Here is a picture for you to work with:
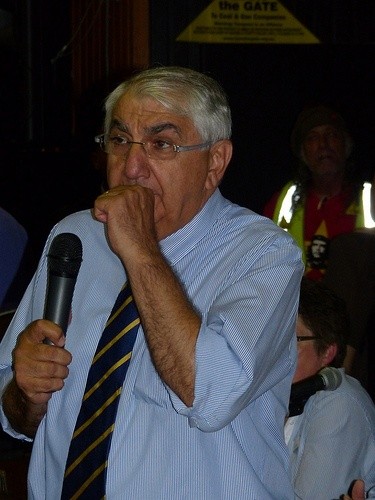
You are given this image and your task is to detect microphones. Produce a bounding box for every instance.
[42,233,83,351]
[285,367,344,418]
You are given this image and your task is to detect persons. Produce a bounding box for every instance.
[0,66,307,500]
[264,105,375,500]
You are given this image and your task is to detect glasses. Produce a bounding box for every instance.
[94,132,222,161]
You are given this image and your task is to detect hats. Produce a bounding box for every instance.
[291,102,345,158]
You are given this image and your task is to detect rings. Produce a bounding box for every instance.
[105,191,110,197]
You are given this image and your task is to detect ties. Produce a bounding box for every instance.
[60,278,142,500]
